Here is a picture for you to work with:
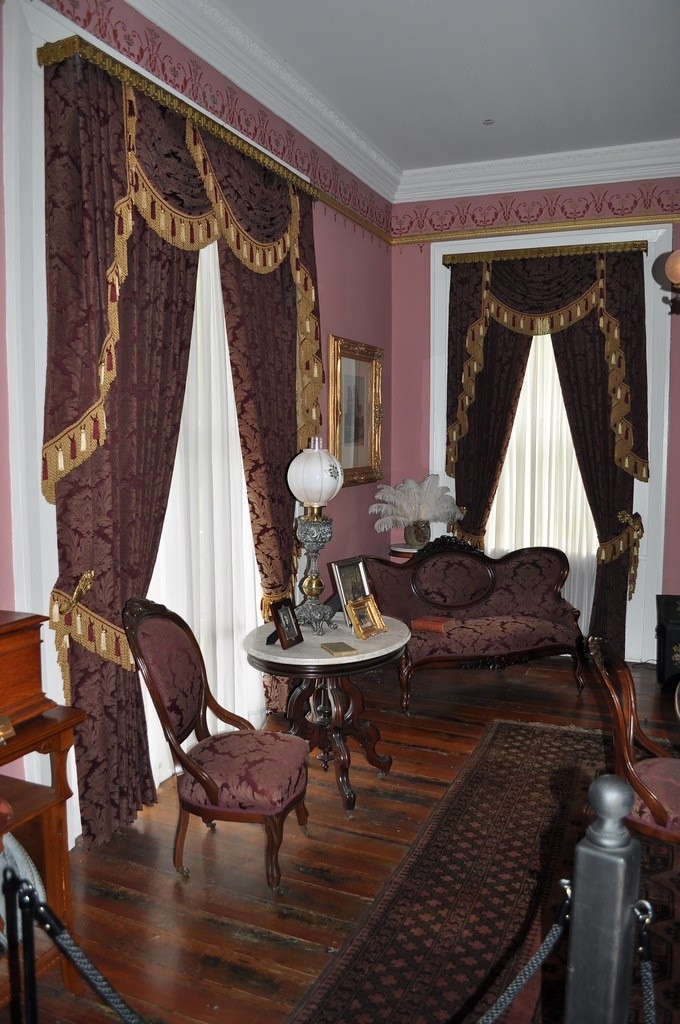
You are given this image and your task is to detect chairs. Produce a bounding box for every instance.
[581,635,680,845]
[122,598,310,896]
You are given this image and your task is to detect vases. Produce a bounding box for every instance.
[404,520,431,549]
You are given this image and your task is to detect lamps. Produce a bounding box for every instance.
[286,449,344,636]
[665,249,680,316]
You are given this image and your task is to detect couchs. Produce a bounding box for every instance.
[362,535,589,718]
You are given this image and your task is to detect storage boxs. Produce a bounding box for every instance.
[0,610,58,746]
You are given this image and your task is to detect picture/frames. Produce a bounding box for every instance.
[345,594,389,640]
[327,334,385,489]
[266,598,304,650]
[330,555,381,628]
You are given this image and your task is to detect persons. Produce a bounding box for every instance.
[344,384,364,442]
[350,576,363,599]
[355,609,369,625]
[282,613,295,639]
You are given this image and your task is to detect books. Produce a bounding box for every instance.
[411,615,456,633]
[322,641,357,656]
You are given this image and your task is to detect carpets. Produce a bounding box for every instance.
[282,719,680,1024]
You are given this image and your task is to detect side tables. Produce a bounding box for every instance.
[388,542,430,558]
[0,703,87,1011]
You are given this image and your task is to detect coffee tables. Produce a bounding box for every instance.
[242,611,411,821]
